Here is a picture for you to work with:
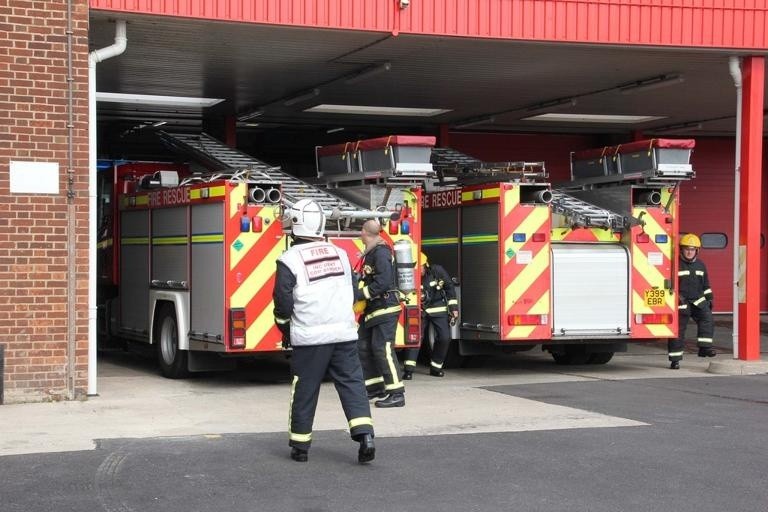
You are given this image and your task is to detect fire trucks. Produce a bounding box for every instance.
[421,137,697,371]
[97,128,439,381]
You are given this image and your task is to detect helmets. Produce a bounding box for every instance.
[680,233,701,248]
[421,252,428,265]
[290,199,326,238]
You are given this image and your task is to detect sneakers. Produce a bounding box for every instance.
[359,434,375,462]
[699,350,715,356]
[430,367,443,376]
[402,372,412,380]
[368,388,386,398]
[292,448,307,462]
[375,393,405,407]
[671,361,679,368]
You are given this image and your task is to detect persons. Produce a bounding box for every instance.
[352,218,406,410]
[402,252,458,379]
[272,197,377,464]
[667,234,717,369]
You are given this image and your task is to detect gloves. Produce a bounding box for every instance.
[358,288,364,300]
[274,318,290,348]
